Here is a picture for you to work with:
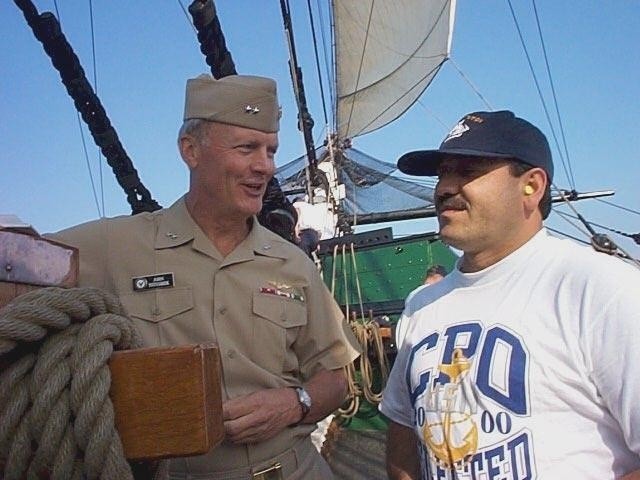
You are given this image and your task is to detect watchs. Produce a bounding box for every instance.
[287,383,313,430]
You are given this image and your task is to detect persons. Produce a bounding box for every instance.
[425,263,445,284]
[376,110,640,480]
[40,72,366,479]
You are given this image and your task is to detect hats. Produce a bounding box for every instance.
[182,72,283,134]
[395,108,555,187]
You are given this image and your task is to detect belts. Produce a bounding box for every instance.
[161,433,314,480]
